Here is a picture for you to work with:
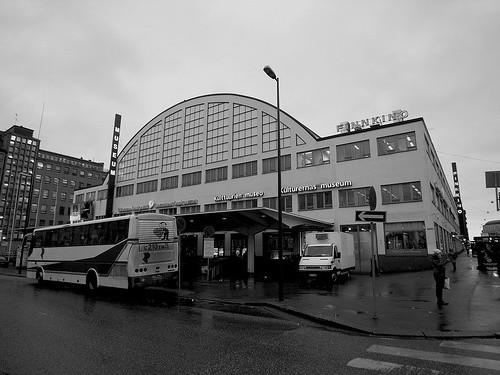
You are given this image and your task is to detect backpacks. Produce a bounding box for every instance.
[451,252,457,260]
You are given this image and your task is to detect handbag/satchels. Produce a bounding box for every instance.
[442,278,451,290]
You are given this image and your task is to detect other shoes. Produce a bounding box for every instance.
[436,301,443,310]
[440,300,449,305]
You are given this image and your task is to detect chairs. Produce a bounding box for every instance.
[58,236,122,246]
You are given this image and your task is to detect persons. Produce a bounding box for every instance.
[432,249,450,305]
[447,248,457,272]
[467,242,470,255]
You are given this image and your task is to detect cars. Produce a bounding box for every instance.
[0,256,8,268]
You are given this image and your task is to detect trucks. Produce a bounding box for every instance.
[296,230,356,283]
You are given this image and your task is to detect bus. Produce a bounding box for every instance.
[14,233,32,268]
[22,213,180,297]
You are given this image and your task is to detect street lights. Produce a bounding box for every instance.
[261,64,284,302]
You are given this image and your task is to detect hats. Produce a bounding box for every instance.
[434,249,442,254]
